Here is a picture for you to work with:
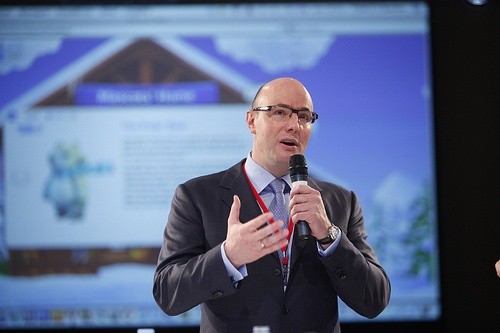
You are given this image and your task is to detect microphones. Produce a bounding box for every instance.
[289,153,311,241]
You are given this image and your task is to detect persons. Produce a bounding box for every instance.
[153,77,391,333]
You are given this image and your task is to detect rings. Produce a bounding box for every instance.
[260,242,265,249]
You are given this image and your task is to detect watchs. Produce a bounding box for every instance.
[317,223,340,244]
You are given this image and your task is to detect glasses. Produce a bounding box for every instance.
[253,106,318,124]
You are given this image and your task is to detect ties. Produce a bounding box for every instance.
[265,179,290,273]
